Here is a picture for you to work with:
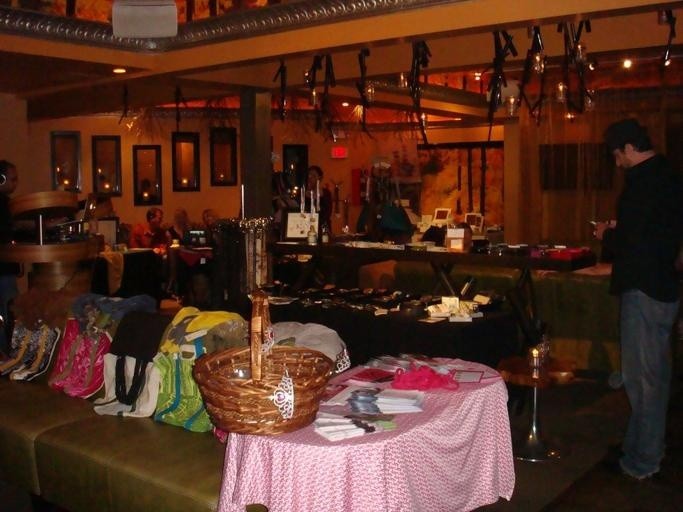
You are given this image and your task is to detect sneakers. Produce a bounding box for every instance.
[8,318,62,387]
[0,317,36,380]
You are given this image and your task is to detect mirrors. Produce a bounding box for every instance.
[282,144,308,195]
[50,127,237,206]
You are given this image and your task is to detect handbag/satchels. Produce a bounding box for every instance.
[151,343,219,434]
[63,280,248,361]
[91,352,163,422]
[44,315,115,405]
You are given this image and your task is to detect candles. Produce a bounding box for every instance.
[241,184,246,222]
[299,180,321,218]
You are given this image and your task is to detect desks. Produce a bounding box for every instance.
[271,286,512,373]
[218,354,517,512]
[499,355,574,463]
[276,245,598,345]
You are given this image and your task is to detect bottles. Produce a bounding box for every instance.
[528,346,549,382]
[170,239,181,248]
[307,225,317,246]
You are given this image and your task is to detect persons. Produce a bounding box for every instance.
[305,165,333,237]
[200,207,236,253]
[125,207,167,250]
[583,116,683,484]
[162,205,198,297]
[0,158,23,355]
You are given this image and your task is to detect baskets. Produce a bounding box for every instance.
[190,292,334,436]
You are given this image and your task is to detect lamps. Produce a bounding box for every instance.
[274,10,676,132]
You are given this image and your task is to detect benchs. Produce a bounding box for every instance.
[34,413,226,512]
[0,375,94,512]
